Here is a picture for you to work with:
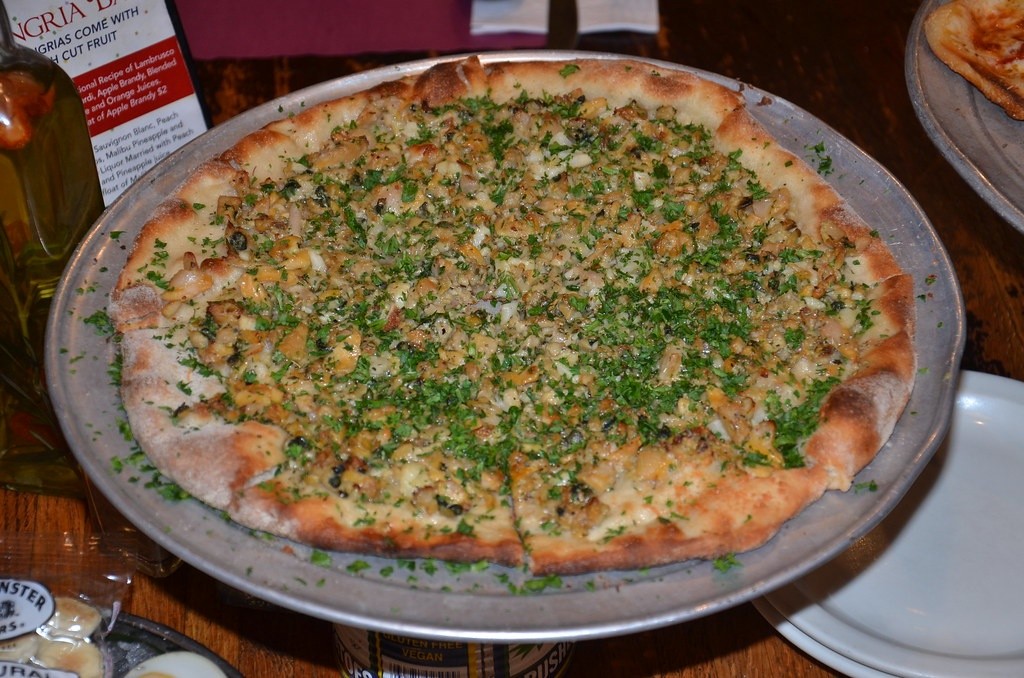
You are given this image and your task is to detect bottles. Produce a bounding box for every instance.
[2,0,205,577]
[328,621,573,677]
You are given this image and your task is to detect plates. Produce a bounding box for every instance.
[749,368,1021,678]
[904,0,1023,239]
[95,610,241,678]
[44,48,967,642]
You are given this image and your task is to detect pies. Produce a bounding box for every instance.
[925,0,1022,123]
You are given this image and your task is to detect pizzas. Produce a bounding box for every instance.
[108,55,918,576]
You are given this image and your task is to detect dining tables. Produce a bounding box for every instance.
[0,40,1021,678]
